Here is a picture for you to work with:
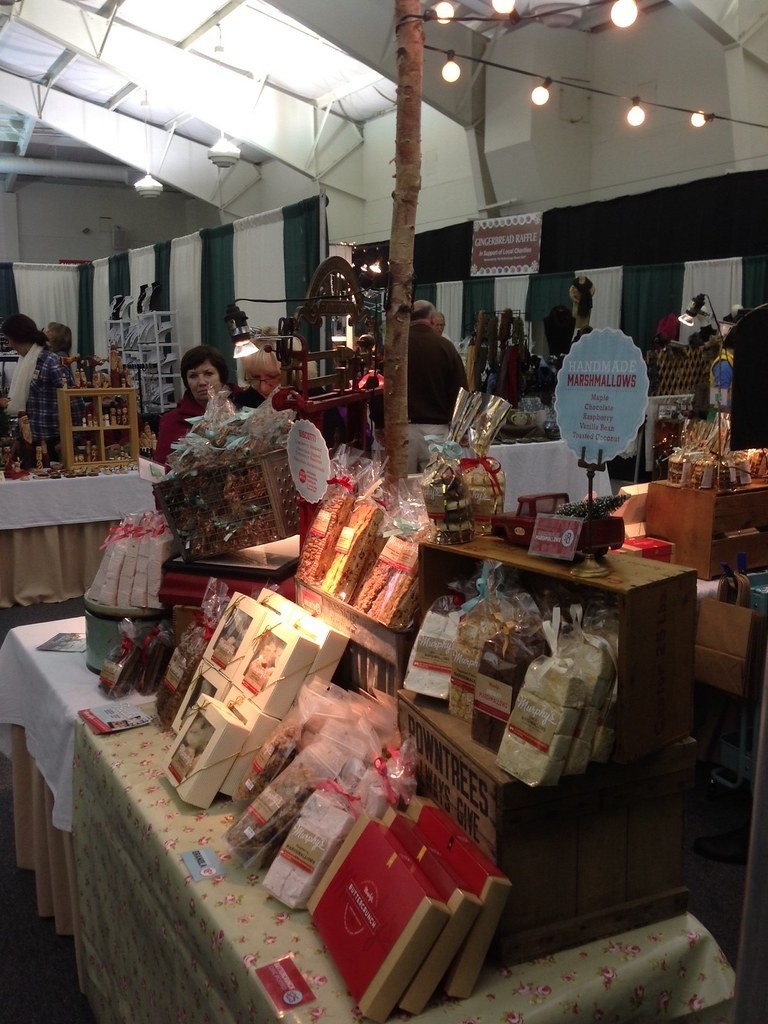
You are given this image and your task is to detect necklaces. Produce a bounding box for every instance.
[108,284,174,401]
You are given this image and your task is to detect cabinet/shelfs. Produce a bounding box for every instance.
[56,387,139,474]
[104,308,182,414]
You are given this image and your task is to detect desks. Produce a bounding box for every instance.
[410,424,612,513]
[0,469,156,609]
[0,616,736,1024]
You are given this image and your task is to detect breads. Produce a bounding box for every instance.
[298,492,423,632]
[424,466,505,545]
[449,613,619,787]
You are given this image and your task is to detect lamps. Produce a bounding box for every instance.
[134,89,163,199]
[360,256,383,273]
[207,22,241,168]
[677,292,708,328]
[222,303,259,359]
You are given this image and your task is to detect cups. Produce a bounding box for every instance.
[510,409,529,426]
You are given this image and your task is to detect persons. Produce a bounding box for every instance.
[0,313,85,461]
[706,349,734,424]
[228,324,348,449]
[45,322,72,374]
[569,272,595,328]
[369,300,471,474]
[435,311,445,335]
[155,344,244,473]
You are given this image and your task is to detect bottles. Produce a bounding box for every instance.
[140,447,153,461]
[82,413,99,427]
[104,407,130,425]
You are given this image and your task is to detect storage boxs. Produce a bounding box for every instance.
[152,446,301,563]
[610,482,649,540]
[294,534,697,1022]
[644,475,768,580]
